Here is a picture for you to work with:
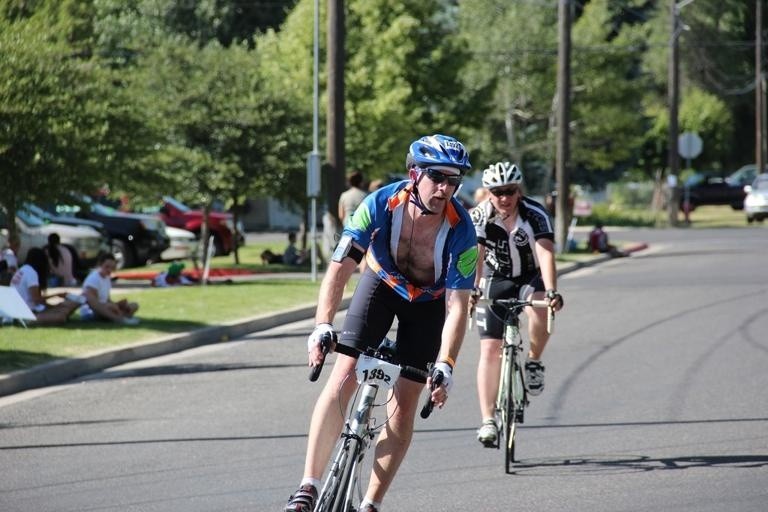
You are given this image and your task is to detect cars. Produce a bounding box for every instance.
[604,163,768,225]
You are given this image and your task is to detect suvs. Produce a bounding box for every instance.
[1,186,247,279]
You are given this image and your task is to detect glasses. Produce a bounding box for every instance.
[416,167,461,186]
[490,189,519,195]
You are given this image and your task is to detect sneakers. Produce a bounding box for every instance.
[476,418,499,442]
[118,315,141,326]
[357,502,380,512]
[524,354,545,396]
[284,484,319,512]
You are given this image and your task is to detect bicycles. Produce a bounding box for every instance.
[467,285,564,475]
[304,334,448,510]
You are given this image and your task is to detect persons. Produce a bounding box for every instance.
[283,232,327,268]
[0,234,140,325]
[467,161,564,447]
[589,223,629,258]
[283,134,477,512]
[368,179,383,193]
[337,170,368,228]
[261,250,284,265]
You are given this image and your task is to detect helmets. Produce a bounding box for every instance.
[481,161,524,191]
[404,133,471,171]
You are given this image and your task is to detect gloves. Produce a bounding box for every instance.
[431,359,453,398]
[544,290,565,309]
[307,323,336,351]
